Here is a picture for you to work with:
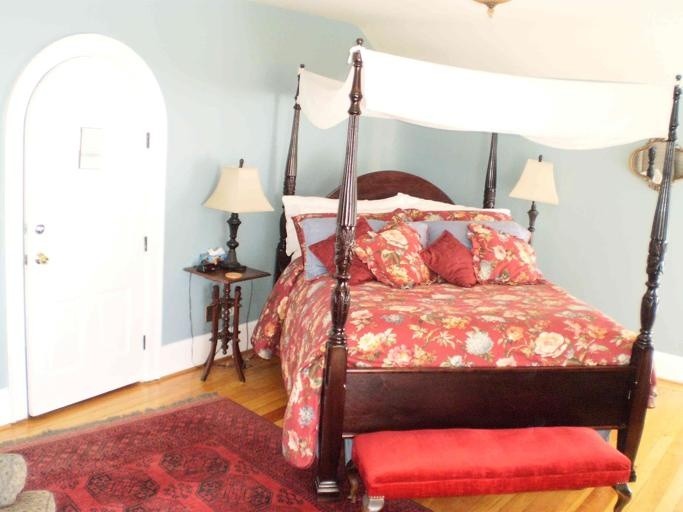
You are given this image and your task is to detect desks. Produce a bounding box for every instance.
[182,265,271,386]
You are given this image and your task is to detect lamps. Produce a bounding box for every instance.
[505,152,559,241]
[200,157,274,270]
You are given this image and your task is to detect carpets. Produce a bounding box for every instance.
[2,392,432,512]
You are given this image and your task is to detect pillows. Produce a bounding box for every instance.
[280,189,541,287]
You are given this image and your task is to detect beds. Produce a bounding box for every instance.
[250,37,683,497]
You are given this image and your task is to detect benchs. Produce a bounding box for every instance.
[346,426,634,512]
